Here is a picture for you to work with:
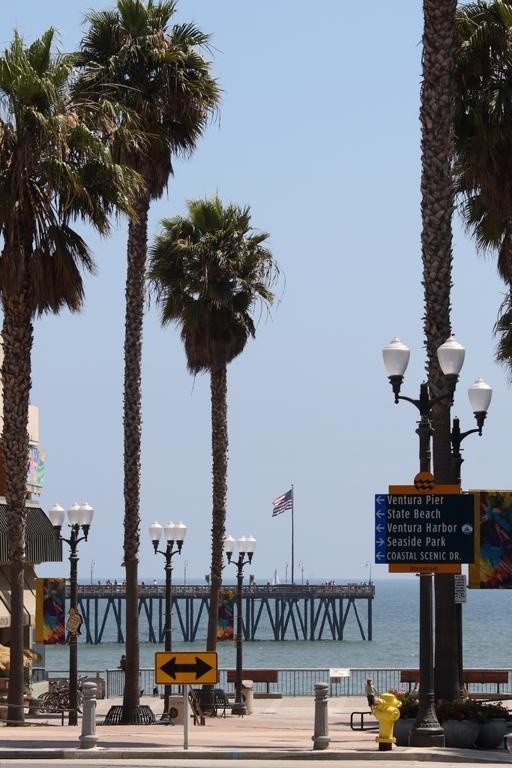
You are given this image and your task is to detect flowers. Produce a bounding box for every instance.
[389,688,508,720]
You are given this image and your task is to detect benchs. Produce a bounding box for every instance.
[189,688,247,718]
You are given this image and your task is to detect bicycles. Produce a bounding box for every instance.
[33,674,89,716]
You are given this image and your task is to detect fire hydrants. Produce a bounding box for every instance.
[370,691,403,752]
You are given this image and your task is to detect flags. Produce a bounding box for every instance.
[272,488,293,518]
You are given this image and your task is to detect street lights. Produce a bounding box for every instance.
[378,329,468,745]
[47,501,95,727]
[146,519,186,722]
[429,377,492,731]
[89,558,95,585]
[222,533,258,717]
[366,559,372,586]
[297,559,304,585]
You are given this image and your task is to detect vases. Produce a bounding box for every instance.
[392,718,507,748]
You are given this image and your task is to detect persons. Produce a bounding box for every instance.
[364,679,377,710]
